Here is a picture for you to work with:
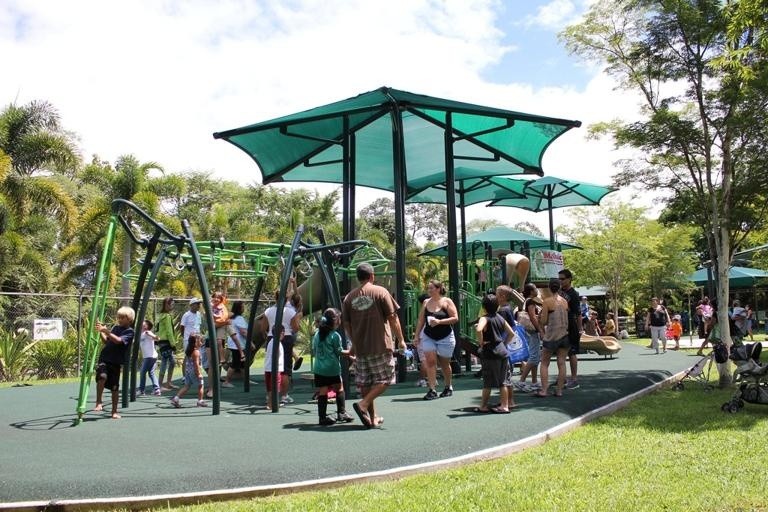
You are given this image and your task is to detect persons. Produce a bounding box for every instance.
[602,293,755,357]
[92,305,135,419]
[311,307,352,425]
[342,263,407,429]
[553,269,583,388]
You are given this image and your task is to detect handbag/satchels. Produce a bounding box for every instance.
[517,298,539,332]
[497,308,530,364]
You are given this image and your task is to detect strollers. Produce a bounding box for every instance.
[672,336,728,396]
[722,341,767,413]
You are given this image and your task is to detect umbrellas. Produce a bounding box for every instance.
[402,166,534,272]
[490,174,624,249]
[416,226,582,284]
[213,87,582,382]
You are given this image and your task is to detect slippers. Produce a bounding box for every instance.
[530,391,552,397]
[472,407,488,412]
[293,357,303,371]
[552,390,562,397]
[491,402,518,414]
[371,416,385,427]
[353,401,371,427]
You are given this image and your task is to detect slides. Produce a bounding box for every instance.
[223,265,330,379]
[575,333,622,360]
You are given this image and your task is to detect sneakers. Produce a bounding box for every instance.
[219,377,235,388]
[170,397,181,408]
[566,380,581,390]
[439,385,453,397]
[530,381,551,390]
[423,390,438,400]
[318,416,337,425]
[338,411,354,422]
[548,380,567,387]
[515,381,533,393]
[206,386,213,398]
[415,378,440,388]
[249,379,260,385]
[196,400,209,407]
[134,389,145,396]
[151,384,180,397]
[264,397,294,410]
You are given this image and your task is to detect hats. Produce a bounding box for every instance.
[671,314,681,321]
[189,297,204,305]
